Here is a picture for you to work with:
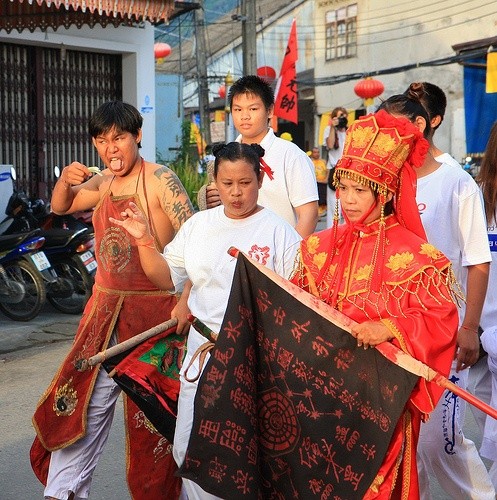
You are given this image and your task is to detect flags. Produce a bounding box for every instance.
[173,249,420,500]
[273,20,299,124]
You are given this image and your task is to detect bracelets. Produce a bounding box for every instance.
[460,325,479,333]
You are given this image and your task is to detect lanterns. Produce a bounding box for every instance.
[217,85,229,99]
[154,42,171,64]
[354,78,385,106]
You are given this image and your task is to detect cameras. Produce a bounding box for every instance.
[336,113,348,128]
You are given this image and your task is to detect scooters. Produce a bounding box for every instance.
[0,186,97,315]
[0,229,52,324]
[37,167,95,250]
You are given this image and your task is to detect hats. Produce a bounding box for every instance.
[333,107,415,194]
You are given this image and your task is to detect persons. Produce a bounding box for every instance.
[108,141,304,500]
[197,77,319,239]
[288,83,497,500]
[28,102,196,500]
